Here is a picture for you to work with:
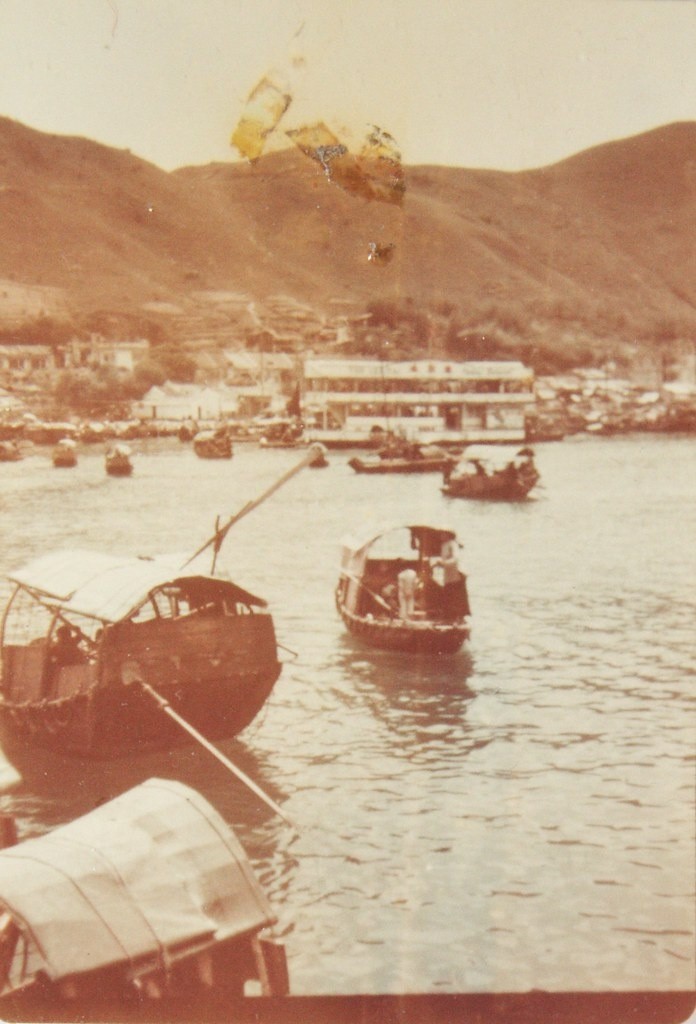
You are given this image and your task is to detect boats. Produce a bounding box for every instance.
[104,445,134,477]
[192,431,236,459]
[1,773,696,1024]
[440,444,541,499]
[0,441,24,460]
[50,439,78,467]
[1,547,281,761]
[261,355,563,446]
[336,511,475,653]
[348,451,458,473]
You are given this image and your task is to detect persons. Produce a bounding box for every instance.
[440,543,471,624]
[470,461,486,475]
[52,624,85,663]
[397,565,416,617]
